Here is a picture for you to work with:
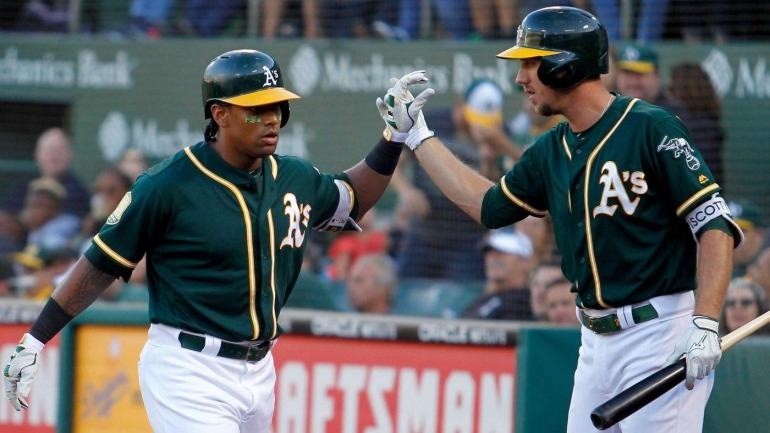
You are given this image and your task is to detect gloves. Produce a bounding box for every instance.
[383,70,435,143]
[3,333,45,411]
[662,314,723,390]
[376,78,435,152]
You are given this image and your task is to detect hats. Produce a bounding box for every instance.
[616,45,660,74]
[7,243,57,271]
[727,199,763,231]
[474,227,534,258]
[462,78,504,129]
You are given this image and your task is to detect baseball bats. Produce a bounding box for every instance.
[589,310,769,431]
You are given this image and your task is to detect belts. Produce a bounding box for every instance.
[179,331,273,363]
[578,304,659,335]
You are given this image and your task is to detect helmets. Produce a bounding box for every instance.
[495,6,609,90]
[201,49,301,128]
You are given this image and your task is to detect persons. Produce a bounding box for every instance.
[0,0,770,338]
[375,7,745,433]
[2,48,435,433]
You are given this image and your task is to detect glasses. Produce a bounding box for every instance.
[724,300,757,308]
